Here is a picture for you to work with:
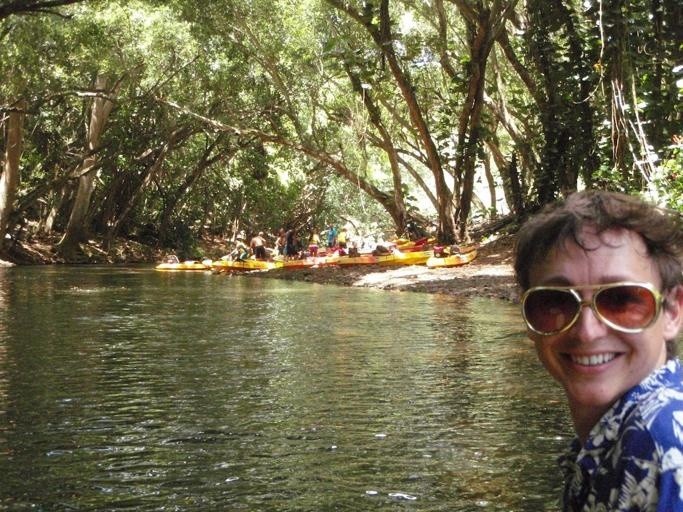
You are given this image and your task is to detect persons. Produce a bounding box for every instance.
[221,224,348,265]
[511,192,682,512]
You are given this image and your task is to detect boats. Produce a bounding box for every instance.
[156,259,208,270]
[200,237,481,280]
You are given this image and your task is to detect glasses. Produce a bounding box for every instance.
[521,281,665,335]
